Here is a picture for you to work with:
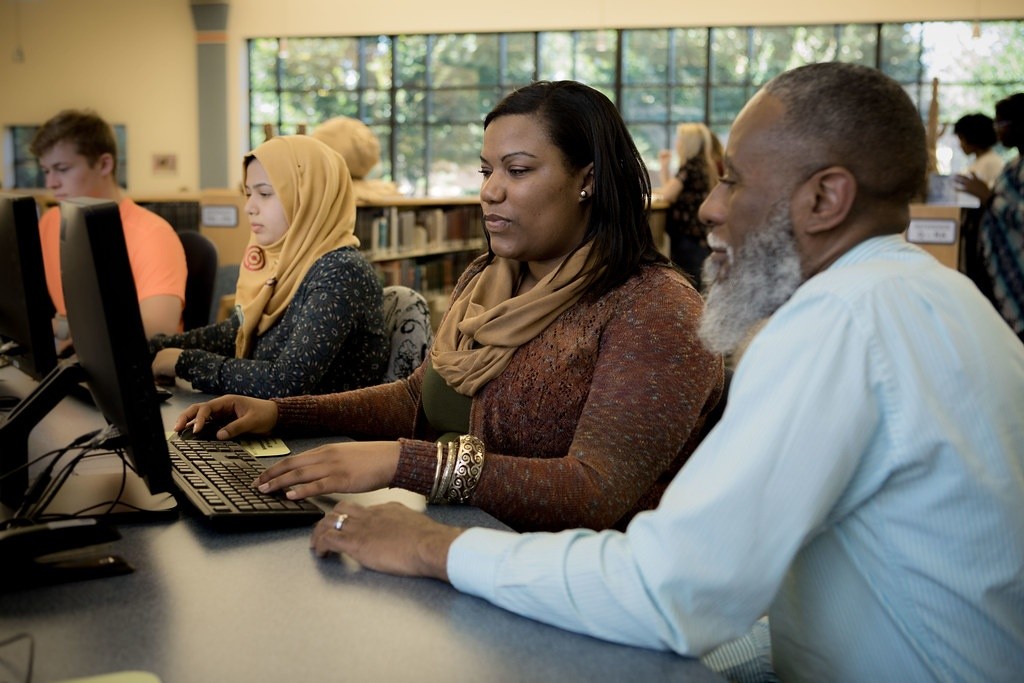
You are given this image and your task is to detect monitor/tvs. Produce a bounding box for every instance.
[0,194,175,505]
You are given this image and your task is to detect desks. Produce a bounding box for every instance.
[1,341,732,683]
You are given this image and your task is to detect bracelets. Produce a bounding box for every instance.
[426,434,485,510]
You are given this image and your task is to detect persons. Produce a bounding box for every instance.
[32,109,187,365]
[150,135,390,408]
[312,59,1024,683]
[951,92,1024,346]
[651,122,725,296]
[172,80,727,562]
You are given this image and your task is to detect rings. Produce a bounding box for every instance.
[335,514,349,530]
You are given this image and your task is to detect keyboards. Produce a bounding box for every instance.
[165,439,327,530]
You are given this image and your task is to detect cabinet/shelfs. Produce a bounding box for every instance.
[351,196,490,333]
